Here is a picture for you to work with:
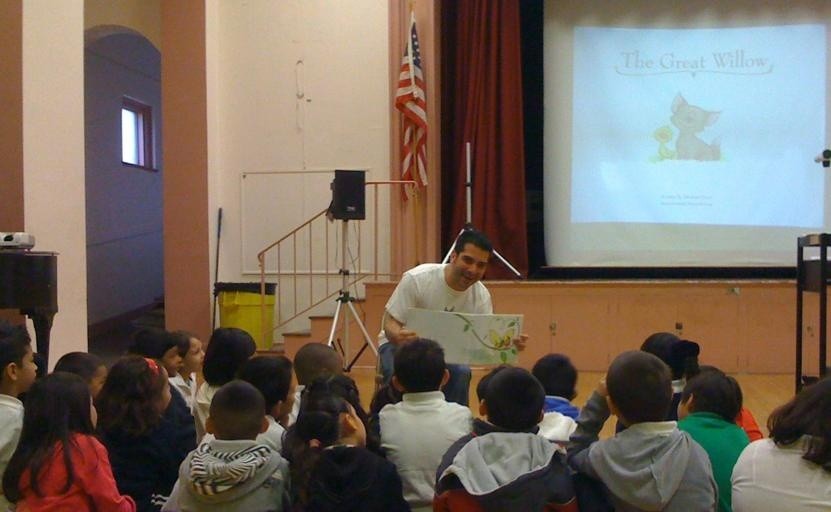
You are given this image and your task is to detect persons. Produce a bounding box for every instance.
[0,322,37,512]
[91,358,174,512]
[734,404,764,444]
[194,355,289,458]
[615,333,702,433]
[131,333,198,451]
[295,342,347,387]
[530,354,582,444]
[370,231,530,455]
[676,367,753,512]
[282,393,409,512]
[433,368,578,511]
[160,382,291,512]
[281,374,382,464]
[1,372,137,512]
[194,327,261,448]
[731,372,831,511]
[379,339,477,512]
[566,351,720,511]
[167,331,207,417]
[52,352,109,403]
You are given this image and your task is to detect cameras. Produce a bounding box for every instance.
[330,169,367,219]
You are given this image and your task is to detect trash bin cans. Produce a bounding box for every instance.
[213,282,277,351]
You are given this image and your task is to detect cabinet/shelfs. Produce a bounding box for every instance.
[1,251,58,375]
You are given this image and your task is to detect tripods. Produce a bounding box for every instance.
[443,144,519,280]
[324,220,380,370]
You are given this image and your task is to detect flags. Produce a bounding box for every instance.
[395,10,429,213]
